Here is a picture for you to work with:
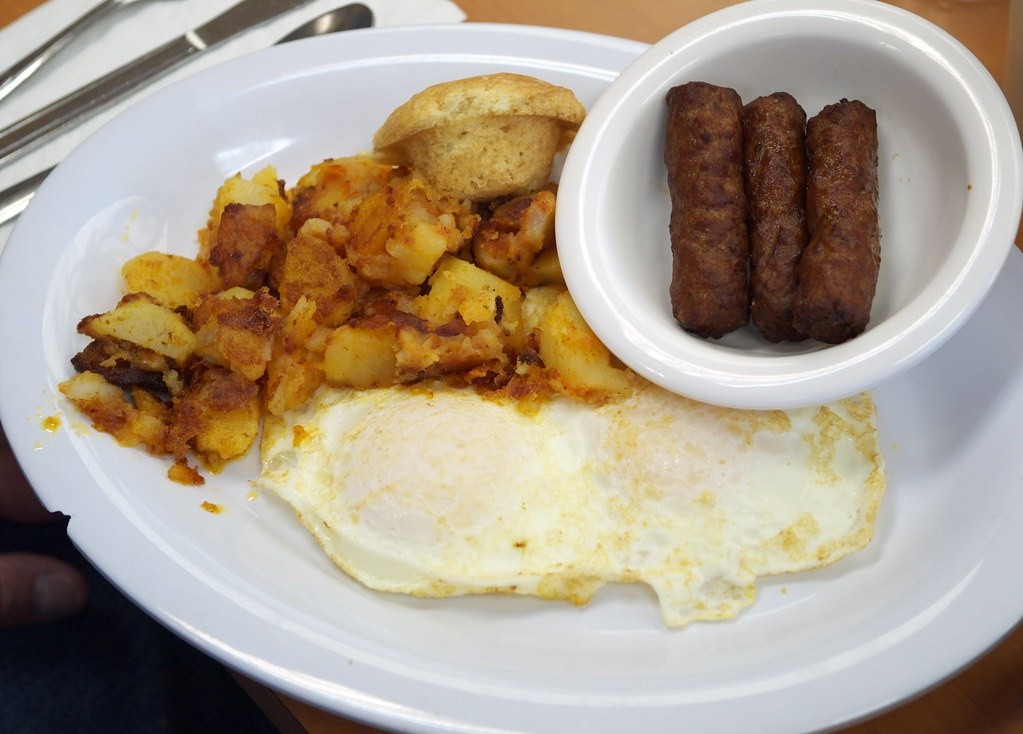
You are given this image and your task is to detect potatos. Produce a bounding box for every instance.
[59,153,622,484]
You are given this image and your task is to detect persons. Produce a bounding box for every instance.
[2,422,281,734]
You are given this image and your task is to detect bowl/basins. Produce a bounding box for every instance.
[548,3,1023,409]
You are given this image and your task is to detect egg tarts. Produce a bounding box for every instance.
[372,74,588,206]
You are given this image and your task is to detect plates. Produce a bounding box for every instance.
[0,20,1022,733]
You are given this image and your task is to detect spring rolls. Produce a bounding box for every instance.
[664,80,883,346]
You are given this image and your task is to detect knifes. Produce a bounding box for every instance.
[0,0,315,158]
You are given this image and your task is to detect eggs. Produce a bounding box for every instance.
[255,370,889,628]
